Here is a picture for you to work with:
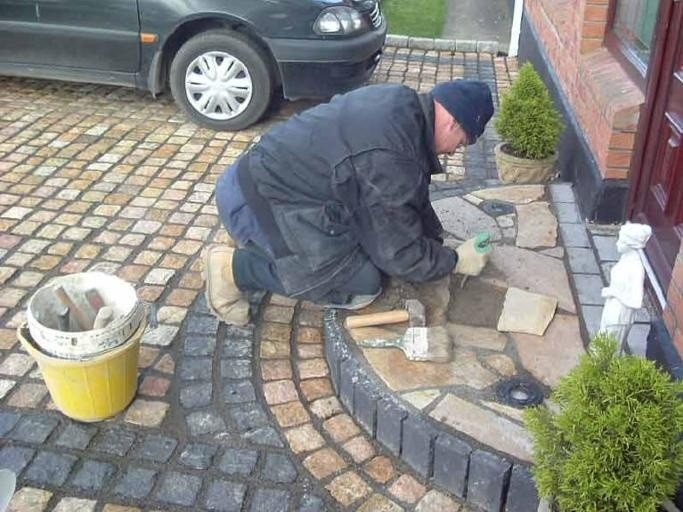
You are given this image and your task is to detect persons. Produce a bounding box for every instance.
[598,222,652,359]
[200,76,495,329]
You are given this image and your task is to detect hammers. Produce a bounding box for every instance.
[347,300,426,328]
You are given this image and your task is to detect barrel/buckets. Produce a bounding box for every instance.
[17,322,147,422]
[28,270,144,357]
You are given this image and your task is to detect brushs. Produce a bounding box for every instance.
[356,326,453,363]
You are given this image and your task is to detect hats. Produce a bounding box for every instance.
[428,76,497,150]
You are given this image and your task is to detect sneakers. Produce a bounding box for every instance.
[198,241,255,330]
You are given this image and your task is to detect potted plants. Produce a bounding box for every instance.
[520,329,683,512]
[494,59,568,186]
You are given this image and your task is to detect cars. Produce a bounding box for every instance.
[0,1,387,131]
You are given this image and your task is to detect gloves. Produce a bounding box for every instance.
[448,230,494,280]
